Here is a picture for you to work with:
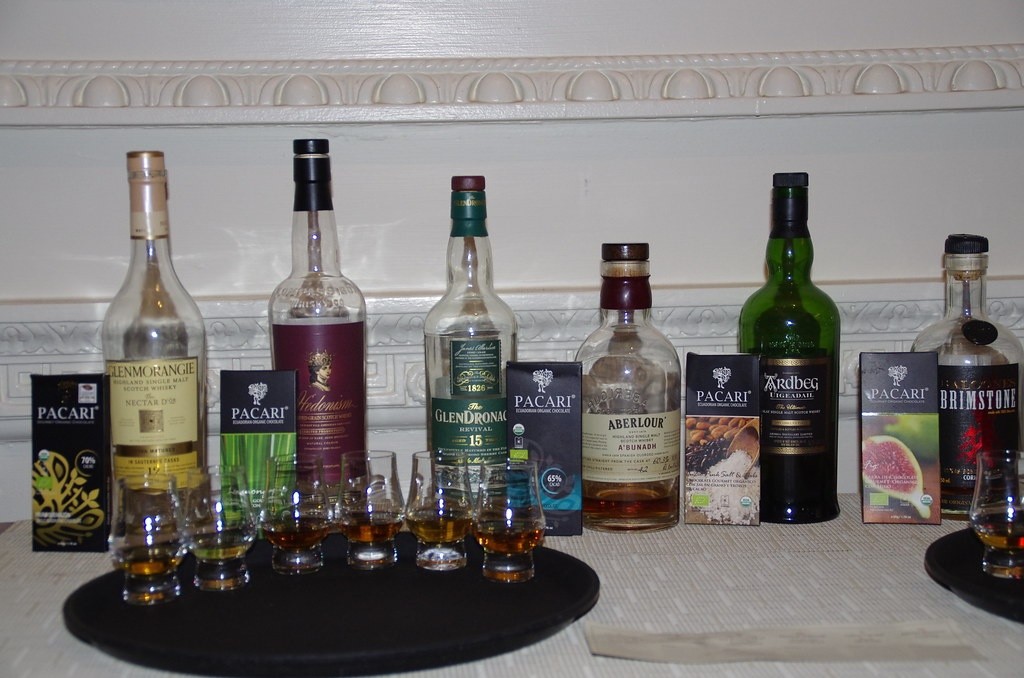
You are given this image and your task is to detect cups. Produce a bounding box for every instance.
[472,459,545,583]
[109,474,186,604]
[970,450,1024,579]
[334,450,405,571]
[184,465,257,591]
[258,454,333,575]
[405,450,471,573]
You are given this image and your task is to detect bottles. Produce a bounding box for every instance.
[103,152,213,548]
[423,176,518,521]
[738,173,840,524]
[269,139,366,534]
[575,244,680,532]
[909,234,1024,514]
